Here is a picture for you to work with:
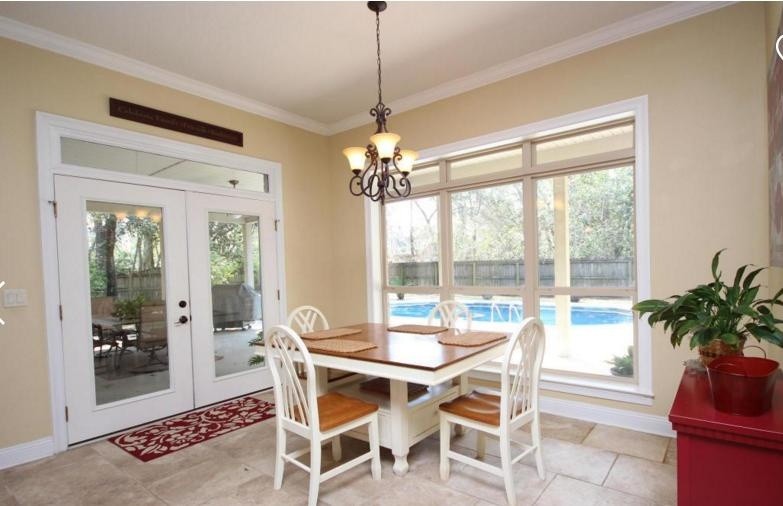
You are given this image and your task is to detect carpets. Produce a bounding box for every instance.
[106,395,277,463]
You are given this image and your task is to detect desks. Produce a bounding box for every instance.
[254,324,524,478]
[91,310,169,371]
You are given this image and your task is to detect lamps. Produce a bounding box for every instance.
[342,1,420,207]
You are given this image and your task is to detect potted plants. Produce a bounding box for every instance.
[631,247,783,369]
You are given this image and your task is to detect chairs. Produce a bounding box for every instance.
[113,302,168,376]
[284,305,368,407]
[90,296,133,361]
[435,315,546,506]
[426,300,472,330]
[262,324,382,506]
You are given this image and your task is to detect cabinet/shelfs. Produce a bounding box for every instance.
[665,358,783,505]
